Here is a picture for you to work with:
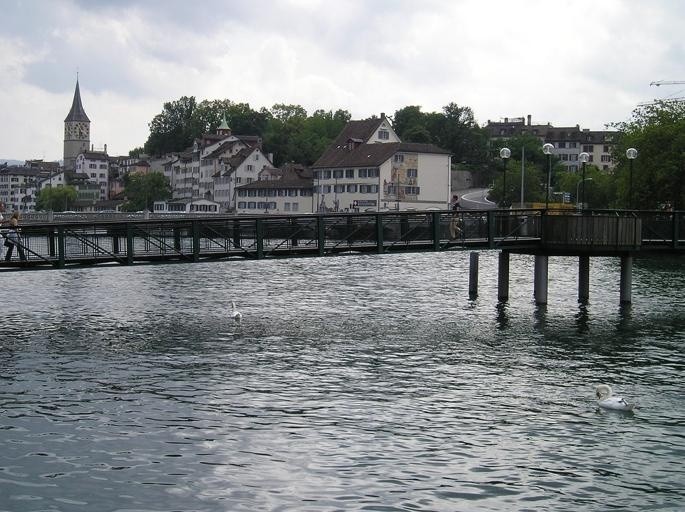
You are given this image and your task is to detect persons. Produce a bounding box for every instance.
[0,201,6,261]
[449,195,465,241]
[4,211,28,261]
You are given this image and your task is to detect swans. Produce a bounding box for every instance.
[596,385,635,412]
[230,301,242,320]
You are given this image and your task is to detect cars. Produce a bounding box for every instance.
[53,210,77,222]
[93,210,123,221]
[126,210,155,220]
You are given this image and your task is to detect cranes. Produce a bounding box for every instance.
[636,80,685,107]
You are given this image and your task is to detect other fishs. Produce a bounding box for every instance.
[596,391,603,399]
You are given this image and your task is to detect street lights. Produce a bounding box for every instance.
[578,152,590,210]
[500,148,511,210]
[626,148,638,210]
[542,143,555,208]
